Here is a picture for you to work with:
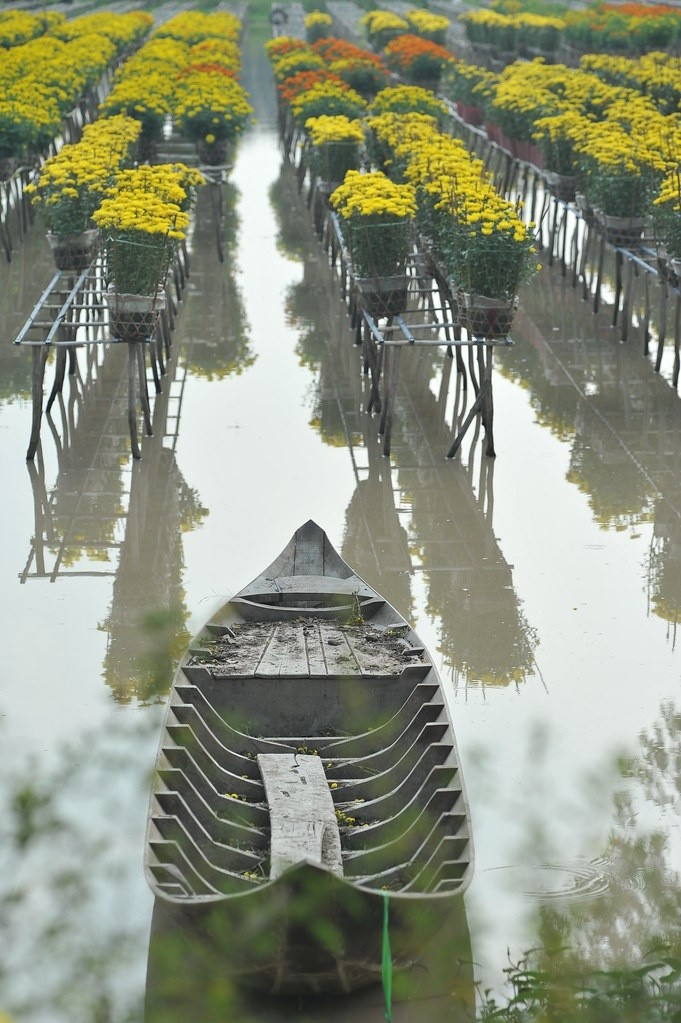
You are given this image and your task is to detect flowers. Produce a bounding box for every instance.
[0,0,681,301]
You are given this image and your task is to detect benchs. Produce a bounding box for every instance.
[233,575,380,603]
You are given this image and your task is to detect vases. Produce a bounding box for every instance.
[319,181,350,211]
[100,289,166,341]
[43,232,98,270]
[549,173,577,200]
[597,213,647,250]
[197,138,228,164]
[656,247,681,289]
[574,193,594,221]
[462,293,519,338]
[530,146,546,169]
[486,126,508,146]
[516,140,528,161]
[458,103,484,125]
[355,273,409,313]
